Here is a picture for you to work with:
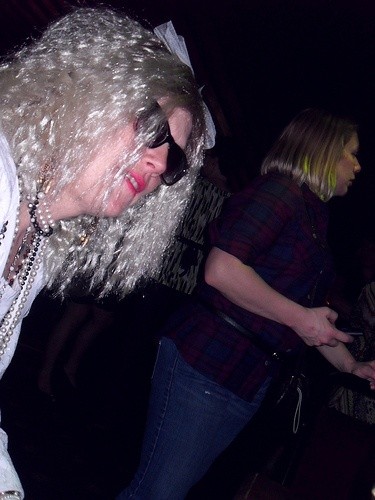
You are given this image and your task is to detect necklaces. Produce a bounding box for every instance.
[0,158,100,356]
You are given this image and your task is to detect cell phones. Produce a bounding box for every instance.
[340,327,364,335]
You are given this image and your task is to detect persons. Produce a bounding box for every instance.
[131,110,374,500]
[0,10,216,500]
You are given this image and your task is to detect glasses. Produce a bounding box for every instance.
[133,97,189,186]
